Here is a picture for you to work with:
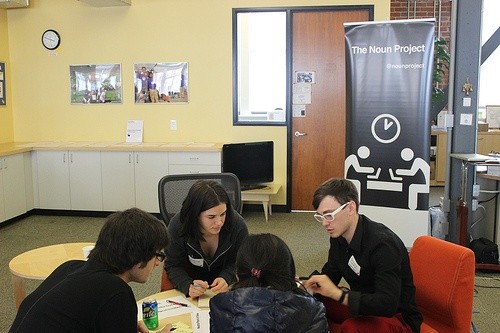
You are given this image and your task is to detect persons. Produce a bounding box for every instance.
[304,178,423,333]
[18,208,173,333]
[135,63,158,103]
[210,233,330,333]
[165,180,248,298]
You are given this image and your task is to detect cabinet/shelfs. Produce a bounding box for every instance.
[430,130,500,186]
[0,150,221,230]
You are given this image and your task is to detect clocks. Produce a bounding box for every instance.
[42,29,60,50]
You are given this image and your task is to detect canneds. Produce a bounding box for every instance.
[142,299,159,330]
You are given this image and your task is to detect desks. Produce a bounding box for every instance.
[9,242,95,310]
[226,182,282,223]
[137,287,230,333]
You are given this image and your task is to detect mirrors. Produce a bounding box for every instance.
[232,7,287,126]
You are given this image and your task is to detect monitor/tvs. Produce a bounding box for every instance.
[223,140,274,191]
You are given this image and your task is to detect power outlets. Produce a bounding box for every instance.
[170,120,177,130]
[472,200,479,211]
[473,184,480,197]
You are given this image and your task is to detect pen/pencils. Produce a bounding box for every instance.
[295,277,309,280]
[170,328,176,331]
[166,300,187,307]
[196,313,199,328]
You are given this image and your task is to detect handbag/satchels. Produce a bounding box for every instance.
[469,237,499,273]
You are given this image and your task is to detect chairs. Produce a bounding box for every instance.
[158,173,242,227]
[409,235,475,333]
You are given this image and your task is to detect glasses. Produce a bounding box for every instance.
[314,202,350,222]
[153,252,166,262]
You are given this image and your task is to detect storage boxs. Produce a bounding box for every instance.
[478,120,488,132]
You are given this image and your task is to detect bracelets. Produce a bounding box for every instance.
[338,292,346,303]
